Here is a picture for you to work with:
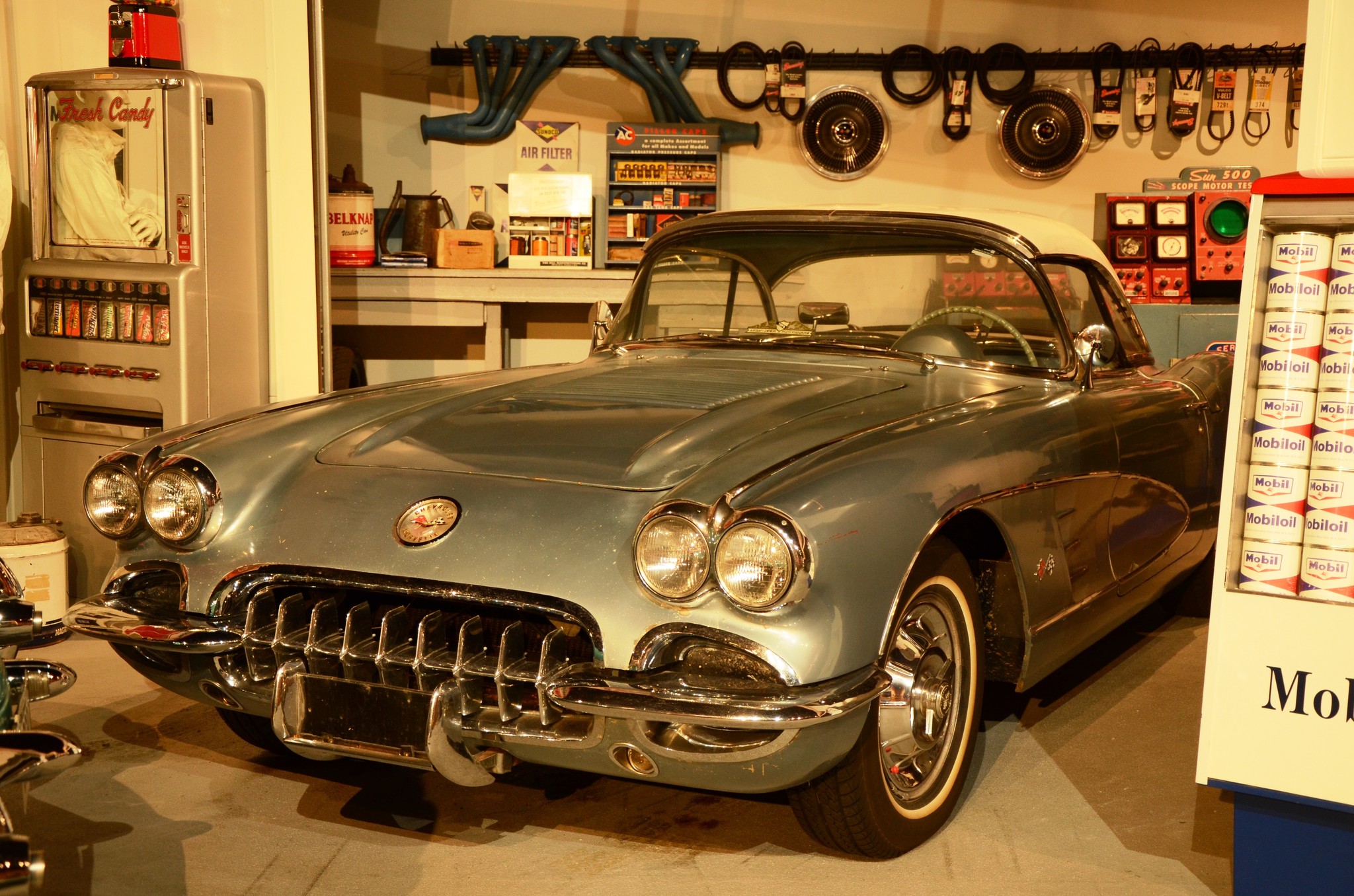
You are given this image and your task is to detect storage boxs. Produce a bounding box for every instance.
[428,225,498,272]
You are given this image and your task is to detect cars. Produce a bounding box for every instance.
[61,210,1234,863]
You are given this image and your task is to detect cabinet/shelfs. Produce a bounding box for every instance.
[599,120,728,268]
[503,177,594,272]
